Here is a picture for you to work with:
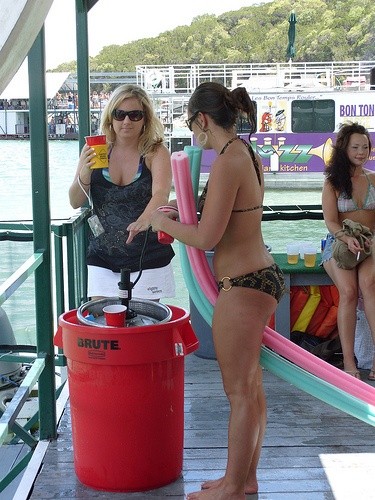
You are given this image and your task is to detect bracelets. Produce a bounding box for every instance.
[80,178,91,185]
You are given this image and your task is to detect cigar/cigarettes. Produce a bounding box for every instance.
[357,251,360,260]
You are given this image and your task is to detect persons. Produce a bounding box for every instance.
[69,84,175,303]
[151,82,285,500]
[318,120,375,380]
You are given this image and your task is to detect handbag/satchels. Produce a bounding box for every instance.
[333,219,373,269]
[291,330,358,369]
[351,309,375,370]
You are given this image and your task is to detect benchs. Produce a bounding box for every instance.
[270,252,335,340]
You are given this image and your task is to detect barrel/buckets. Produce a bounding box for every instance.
[190,244,272,360]
[53,304,200,493]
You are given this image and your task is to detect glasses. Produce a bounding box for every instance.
[112,109,144,121]
[185,112,205,132]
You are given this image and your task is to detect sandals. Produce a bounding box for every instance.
[368,370,375,381]
[344,370,361,382]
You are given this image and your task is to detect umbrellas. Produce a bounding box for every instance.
[286,13,298,80]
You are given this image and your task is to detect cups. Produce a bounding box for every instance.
[156,205,179,245]
[84,135,106,146]
[87,145,108,169]
[321,239,327,256]
[103,305,127,328]
[285,243,300,264]
[300,241,312,259]
[304,247,317,268]
